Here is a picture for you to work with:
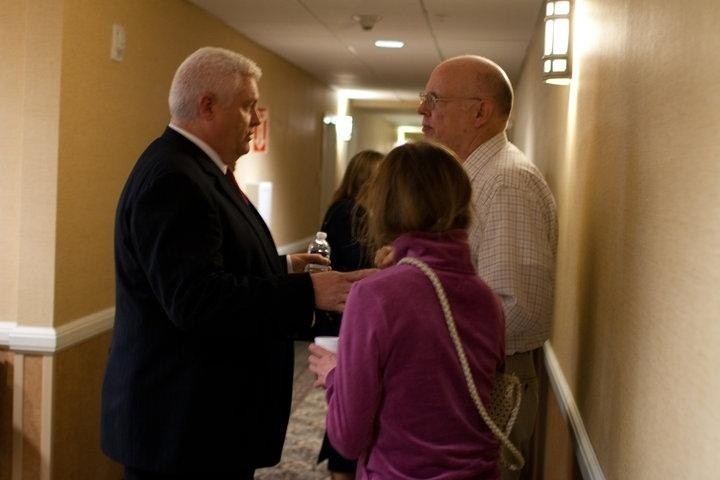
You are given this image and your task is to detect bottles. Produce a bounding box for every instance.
[306,231,331,273]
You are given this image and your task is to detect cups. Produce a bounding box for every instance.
[314,336,338,355]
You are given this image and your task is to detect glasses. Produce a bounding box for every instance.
[416,87,483,111]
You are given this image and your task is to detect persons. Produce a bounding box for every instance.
[418,55,559,480]
[308,138,505,480]
[102,46,380,480]
[307,150,385,342]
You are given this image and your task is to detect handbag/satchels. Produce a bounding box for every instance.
[483,370,525,442]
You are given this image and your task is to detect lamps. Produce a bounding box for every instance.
[537,0,576,86]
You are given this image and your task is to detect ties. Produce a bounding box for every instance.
[224,164,250,207]
